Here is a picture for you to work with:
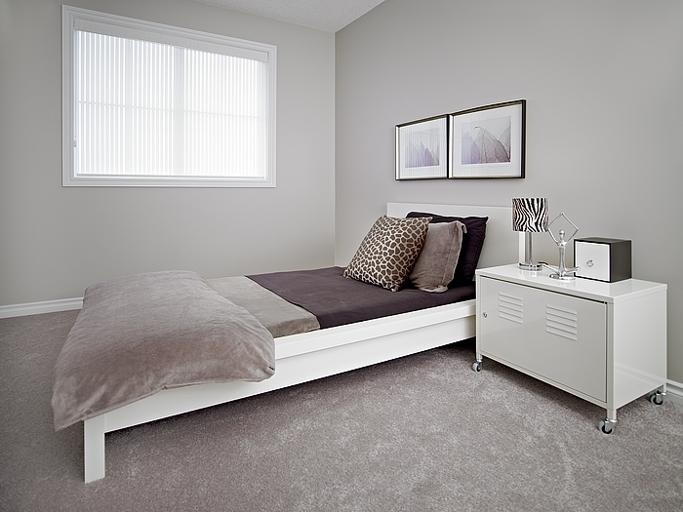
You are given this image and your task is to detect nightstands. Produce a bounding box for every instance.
[474,263,670,411]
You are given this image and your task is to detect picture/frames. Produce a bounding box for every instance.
[393,99,529,183]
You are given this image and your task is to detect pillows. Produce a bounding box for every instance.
[342,211,489,295]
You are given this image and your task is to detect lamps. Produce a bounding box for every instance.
[510,194,548,271]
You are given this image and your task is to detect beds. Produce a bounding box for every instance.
[72,200,525,485]
